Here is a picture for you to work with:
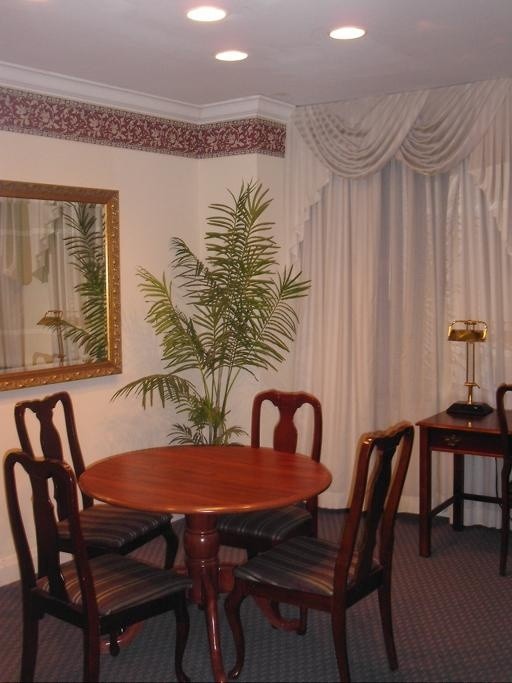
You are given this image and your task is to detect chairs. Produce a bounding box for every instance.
[250,389,323,629]
[12,391,180,579]
[2,449,195,682]
[225,421,417,682]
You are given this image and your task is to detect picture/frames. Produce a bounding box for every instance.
[0,179,124,391]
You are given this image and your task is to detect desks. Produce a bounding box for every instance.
[414,406,511,575]
